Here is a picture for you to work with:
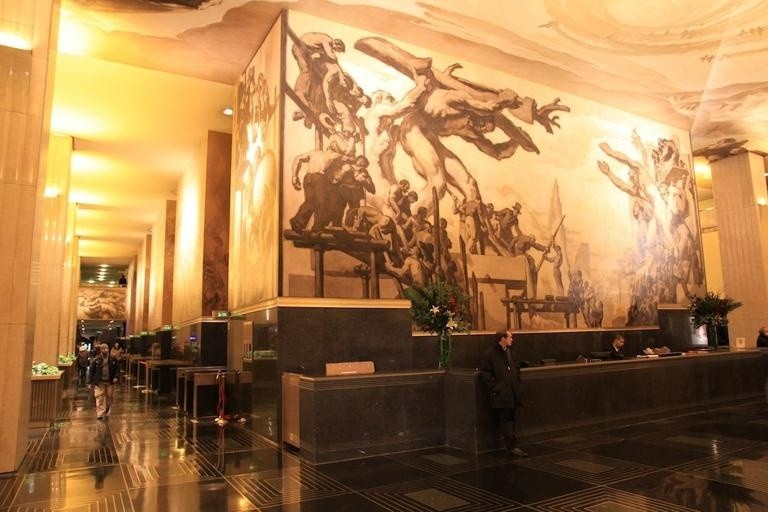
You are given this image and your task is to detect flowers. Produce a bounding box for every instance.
[33,353,76,379]
[408,277,473,352]
[688,287,743,334]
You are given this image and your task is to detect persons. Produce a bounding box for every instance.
[757,327,768,347]
[86,344,120,420]
[606,335,625,352]
[78,341,124,382]
[481,329,528,456]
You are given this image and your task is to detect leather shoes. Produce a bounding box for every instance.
[511,445,530,458]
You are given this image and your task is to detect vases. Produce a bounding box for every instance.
[439,332,451,367]
[707,326,721,346]
